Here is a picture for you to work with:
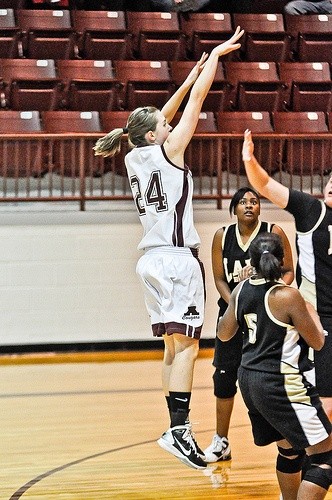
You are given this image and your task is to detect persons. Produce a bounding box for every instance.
[92,24,244,471]
[202,187,294,463]
[217,234,332,500]
[242,129,332,424]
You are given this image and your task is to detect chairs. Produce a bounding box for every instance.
[0,0,332,177]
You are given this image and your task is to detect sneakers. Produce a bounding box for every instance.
[156,428,207,469]
[200,435,231,463]
